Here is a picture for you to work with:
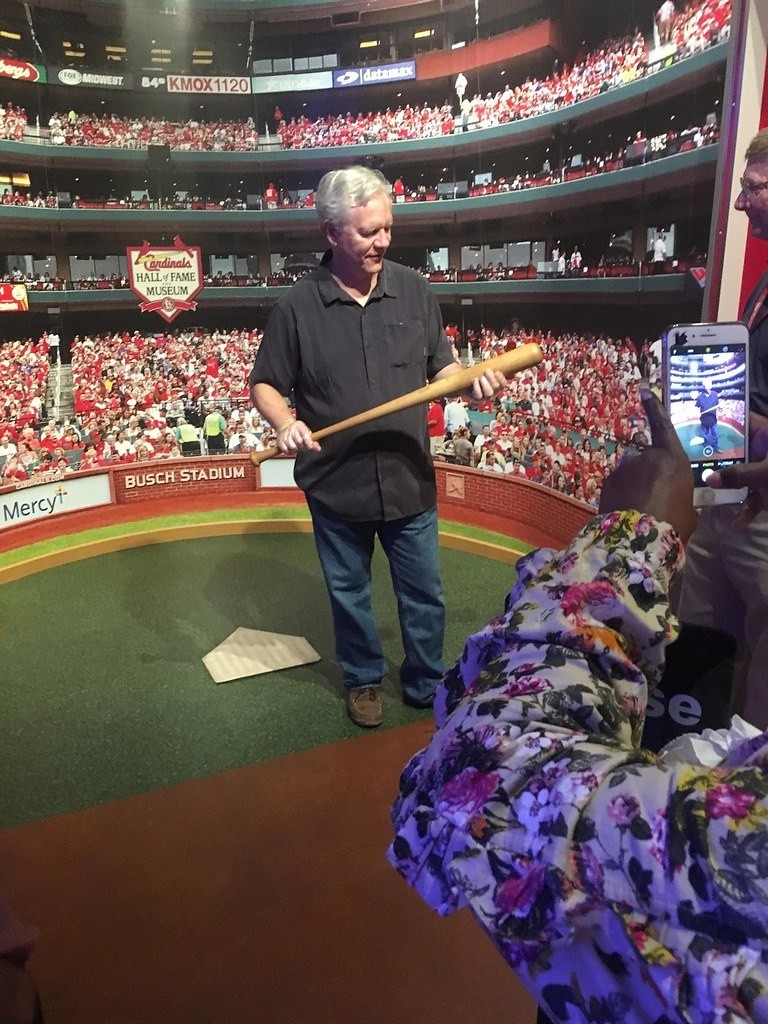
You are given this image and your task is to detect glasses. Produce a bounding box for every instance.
[741,176,768,197]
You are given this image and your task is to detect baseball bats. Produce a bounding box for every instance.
[249,341,544,468]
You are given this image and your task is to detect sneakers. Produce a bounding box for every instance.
[347,688,383,726]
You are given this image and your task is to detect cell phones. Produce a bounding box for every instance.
[662,321,750,509]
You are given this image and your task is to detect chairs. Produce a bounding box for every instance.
[429,261,700,282]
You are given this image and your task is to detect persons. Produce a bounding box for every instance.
[249,165,508,728]
[0,0,732,510]
[387,388,768,1024]
[695,380,723,454]
[677,130,768,718]
